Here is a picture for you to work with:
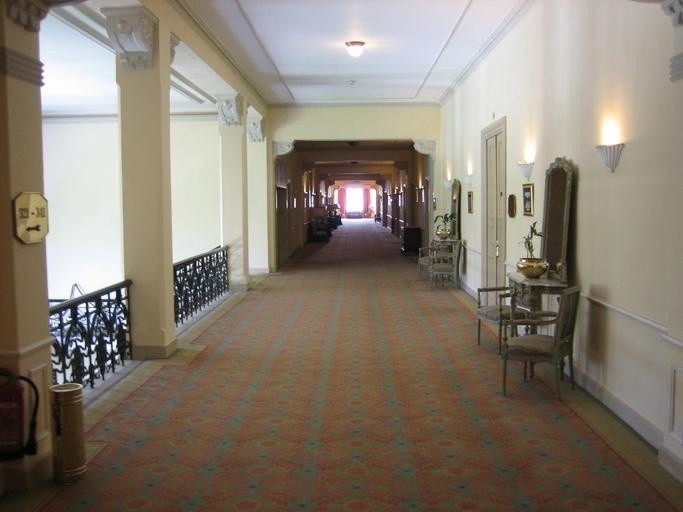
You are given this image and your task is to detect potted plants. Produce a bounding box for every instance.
[434,213,456,239]
[516,221,548,278]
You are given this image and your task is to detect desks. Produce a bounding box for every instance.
[507,273,569,380]
[432,236,458,287]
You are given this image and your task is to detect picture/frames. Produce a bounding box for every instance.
[467,192,473,212]
[521,183,534,216]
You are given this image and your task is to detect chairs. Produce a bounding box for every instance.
[476,286,529,353]
[418,246,436,274]
[501,285,582,401]
[430,240,462,290]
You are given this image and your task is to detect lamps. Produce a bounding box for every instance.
[517,162,533,180]
[596,143,625,172]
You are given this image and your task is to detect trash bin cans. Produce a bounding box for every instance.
[49,383,87,484]
[401,226,421,255]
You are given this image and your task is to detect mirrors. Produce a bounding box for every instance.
[450,178,461,240]
[540,156,574,283]
